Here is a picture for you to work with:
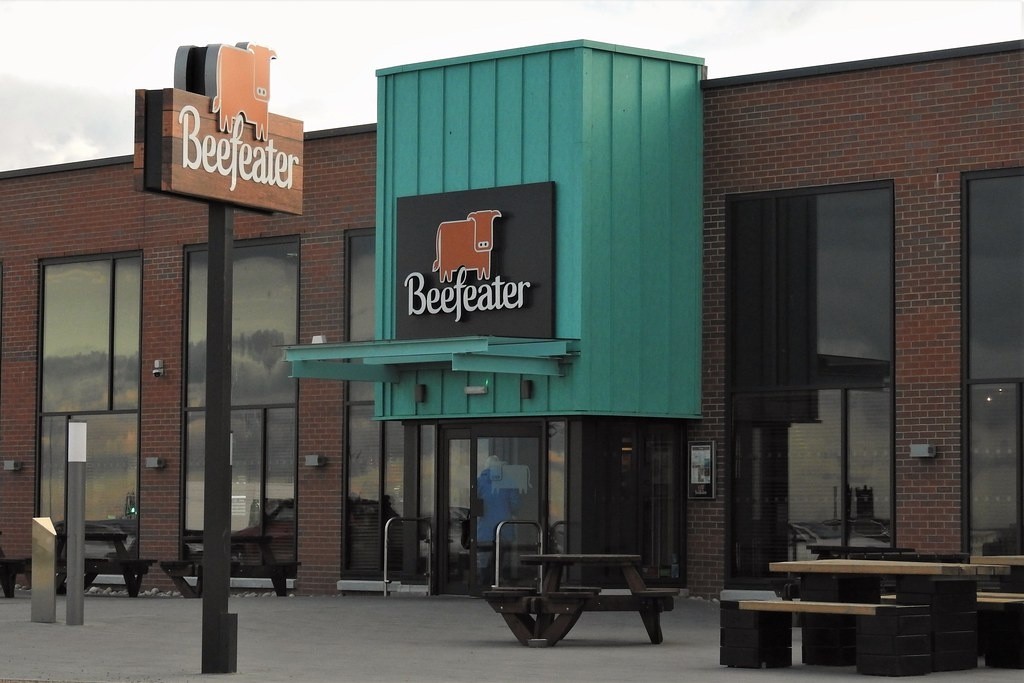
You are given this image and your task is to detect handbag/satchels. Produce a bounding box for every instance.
[461,511,470,550]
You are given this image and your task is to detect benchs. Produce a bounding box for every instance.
[482,586,679,647]
[0,557,301,598]
[720,591,1024,677]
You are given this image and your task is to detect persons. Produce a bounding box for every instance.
[477,455,518,589]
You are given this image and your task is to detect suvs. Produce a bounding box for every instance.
[232,498,405,571]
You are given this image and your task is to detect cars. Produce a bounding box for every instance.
[787,523,891,561]
[50,521,136,560]
[185,543,203,556]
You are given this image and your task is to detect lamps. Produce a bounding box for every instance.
[415,385,426,402]
[152,360,165,377]
[522,379,532,399]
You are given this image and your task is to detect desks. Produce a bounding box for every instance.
[519,554,662,647]
[178,533,285,598]
[55,533,138,598]
[769,545,1024,677]
